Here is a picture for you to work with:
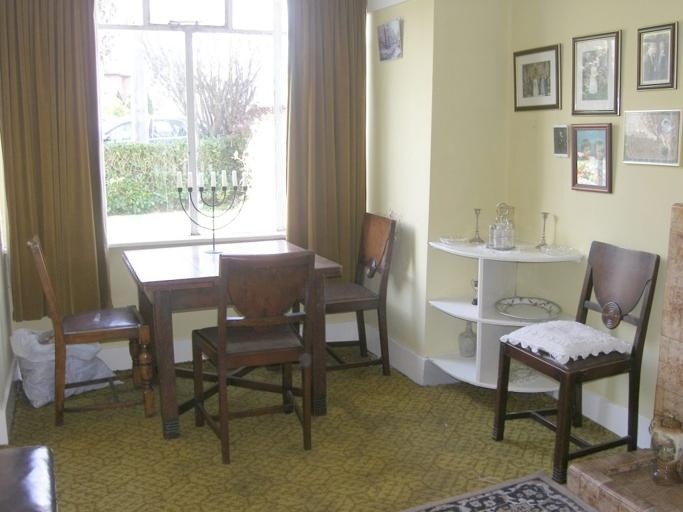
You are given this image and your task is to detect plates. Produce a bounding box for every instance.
[494,297,562,321]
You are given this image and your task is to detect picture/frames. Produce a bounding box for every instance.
[568,123,614,194]
[509,42,563,114]
[571,28,622,116]
[550,125,568,159]
[621,108,682,167]
[635,20,680,91]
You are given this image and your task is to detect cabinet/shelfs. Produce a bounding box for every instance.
[422,237,587,396]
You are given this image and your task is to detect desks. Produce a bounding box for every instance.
[116,235,344,443]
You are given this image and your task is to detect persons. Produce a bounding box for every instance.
[643,40,667,81]
[524,75,550,96]
[577,138,606,186]
[558,129,567,153]
[589,58,600,95]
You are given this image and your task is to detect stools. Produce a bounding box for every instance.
[0,442,57,512]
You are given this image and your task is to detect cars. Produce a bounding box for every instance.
[102,113,186,142]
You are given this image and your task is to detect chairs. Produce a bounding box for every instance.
[287,206,399,378]
[489,235,661,487]
[181,247,319,465]
[22,232,157,430]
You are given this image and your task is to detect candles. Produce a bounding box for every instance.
[173,167,249,189]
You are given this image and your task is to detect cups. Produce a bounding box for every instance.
[488,223,514,249]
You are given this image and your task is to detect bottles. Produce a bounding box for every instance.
[457,320,477,358]
[651,410,682,487]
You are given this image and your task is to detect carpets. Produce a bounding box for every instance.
[388,468,605,512]
